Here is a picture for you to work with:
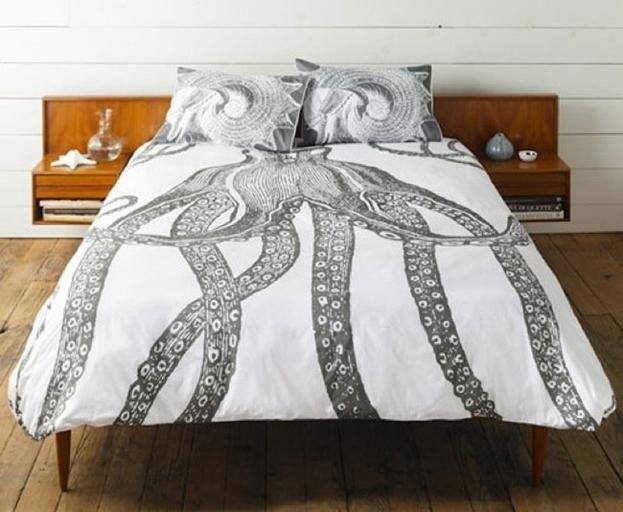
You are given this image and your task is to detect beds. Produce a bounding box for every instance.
[8,94,618,491]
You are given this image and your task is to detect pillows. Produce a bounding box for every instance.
[152,66,310,154]
[296,58,443,145]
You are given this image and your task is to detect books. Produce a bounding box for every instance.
[39,200,102,222]
[502,196,564,220]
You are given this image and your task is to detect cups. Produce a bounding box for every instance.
[518,150,537,162]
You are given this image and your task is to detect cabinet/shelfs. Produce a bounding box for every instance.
[474,152,571,223]
[31,153,132,226]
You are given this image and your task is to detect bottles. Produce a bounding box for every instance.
[485,131,514,161]
